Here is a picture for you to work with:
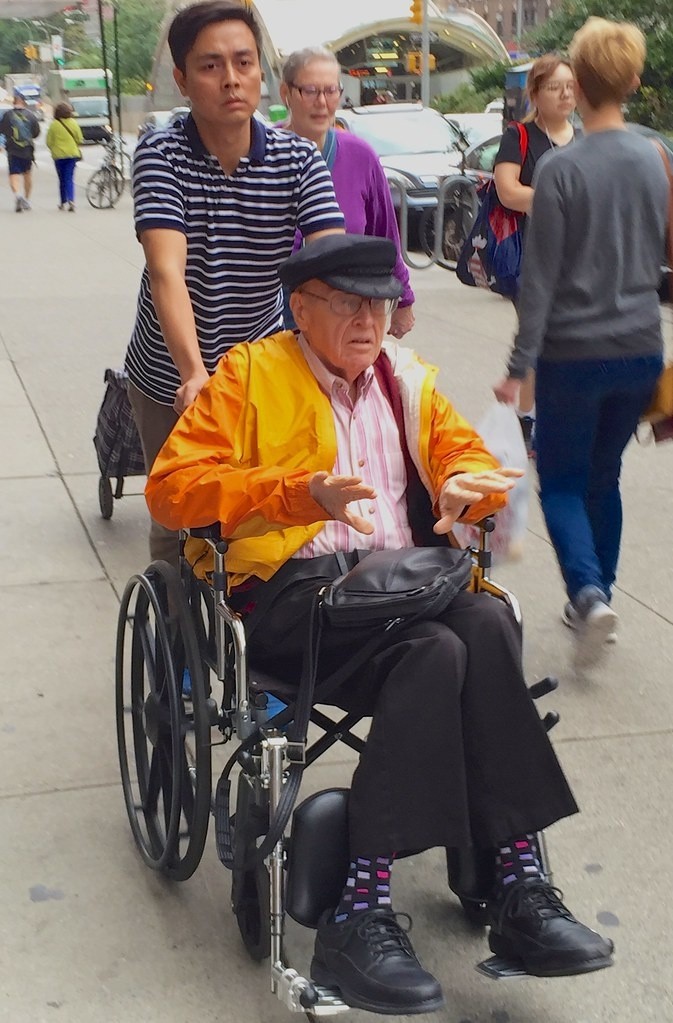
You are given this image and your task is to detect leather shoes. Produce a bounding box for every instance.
[487,879,614,975]
[307,914,440,1012]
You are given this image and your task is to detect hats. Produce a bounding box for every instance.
[277,224,403,299]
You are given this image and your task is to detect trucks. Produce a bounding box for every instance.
[54,66,105,141]
[5,73,43,118]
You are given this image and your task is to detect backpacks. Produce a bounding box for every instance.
[8,108,34,148]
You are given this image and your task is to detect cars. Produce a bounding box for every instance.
[331,101,473,227]
[438,100,673,202]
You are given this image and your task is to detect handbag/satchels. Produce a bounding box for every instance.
[636,367,673,441]
[456,119,531,301]
[321,541,474,624]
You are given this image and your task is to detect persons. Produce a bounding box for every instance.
[491,14,673,678]
[124,0,345,720]
[46,102,84,210]
[495,55,584,458]
[0,93,40,212]
[145,233,614,1014]
[270,48,416,340]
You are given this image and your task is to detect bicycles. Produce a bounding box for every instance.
[86,133,130,211]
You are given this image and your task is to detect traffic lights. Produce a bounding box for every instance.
[408,0,424,25]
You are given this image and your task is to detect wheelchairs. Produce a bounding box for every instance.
[112,515,615,1022]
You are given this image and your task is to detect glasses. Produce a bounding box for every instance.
[297,285,401,315]
[539,81,578,95]
[287,79,344,103]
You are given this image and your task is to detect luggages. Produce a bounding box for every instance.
[89,367,165,521]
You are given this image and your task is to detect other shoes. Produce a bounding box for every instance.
[15,196,23,211]
[68,201,74,211]
[23,198,32,211]
[58,205,65,210]
[559,587,620,676]
